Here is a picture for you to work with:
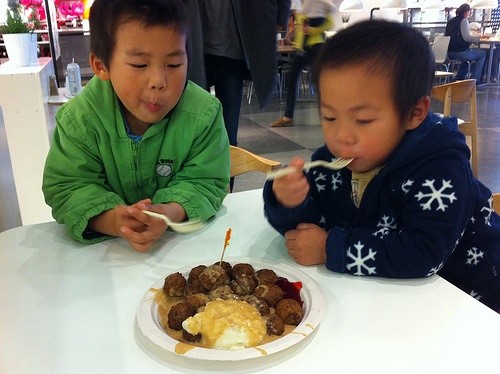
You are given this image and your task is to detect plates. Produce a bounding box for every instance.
[137,256,325,361]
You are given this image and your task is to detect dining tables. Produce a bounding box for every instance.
[1,187,500,374]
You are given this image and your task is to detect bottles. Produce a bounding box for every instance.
[66,58,82,99]
[49,74,59,96]
[65,72,71,97]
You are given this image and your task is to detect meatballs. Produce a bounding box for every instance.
[163,261,304,344]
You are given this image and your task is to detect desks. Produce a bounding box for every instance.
[478,38,500,83]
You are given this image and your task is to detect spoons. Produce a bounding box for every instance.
[141,209,203,233]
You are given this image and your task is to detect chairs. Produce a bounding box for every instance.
[433,33,478,178]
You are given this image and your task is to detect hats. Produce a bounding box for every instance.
[457,3,470,13]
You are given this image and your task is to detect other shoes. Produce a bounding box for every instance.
[271,118,294,127]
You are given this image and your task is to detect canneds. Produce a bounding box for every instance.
[67,63,82,95]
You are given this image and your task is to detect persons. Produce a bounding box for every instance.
[445,4,500,85]
[41,0,231,254]
[185,0,292,192]
[272,0,338,125]
[262,18,500,314]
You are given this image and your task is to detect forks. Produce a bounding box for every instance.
[274,157,357,180]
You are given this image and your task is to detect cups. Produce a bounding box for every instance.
[276,33,281,39]
[276,38,284,48]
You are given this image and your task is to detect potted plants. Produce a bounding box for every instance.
[0,6,41,68]
